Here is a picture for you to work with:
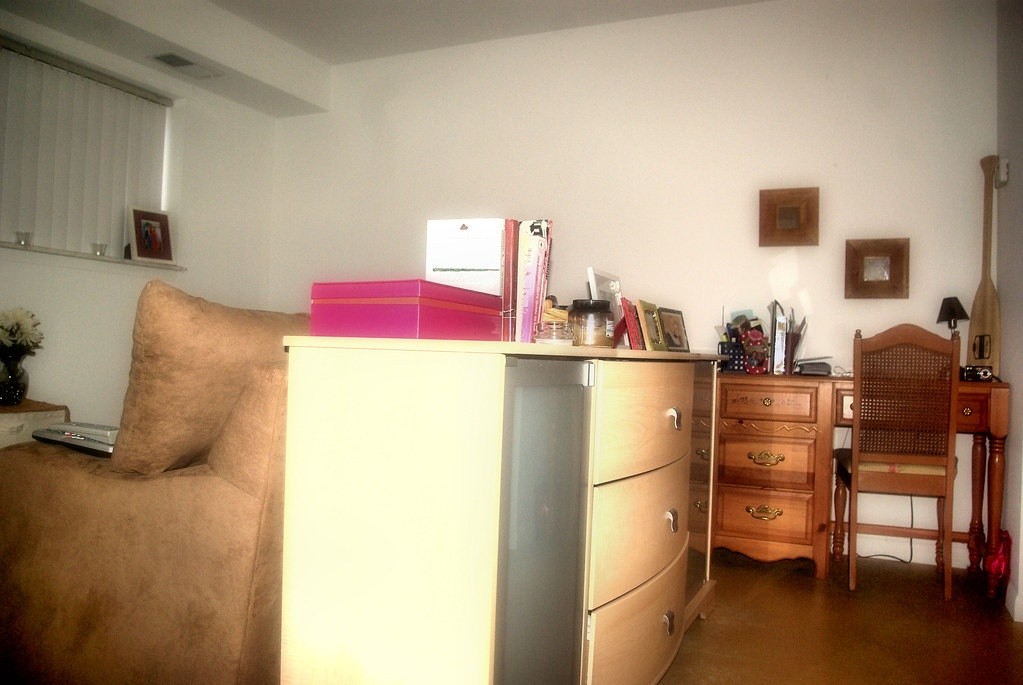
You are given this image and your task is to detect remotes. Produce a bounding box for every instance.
[48,422,119,445]
[32,428,114,459]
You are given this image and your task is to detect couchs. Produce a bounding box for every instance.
[0,367,289,685]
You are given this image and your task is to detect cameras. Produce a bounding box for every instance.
[964,365,993,383]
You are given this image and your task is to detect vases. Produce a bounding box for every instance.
[0,357,28,407]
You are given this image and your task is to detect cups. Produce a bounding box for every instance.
[534,322,575,340]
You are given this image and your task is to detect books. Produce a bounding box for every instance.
[425,218,553,343]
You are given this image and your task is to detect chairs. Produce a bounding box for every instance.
[833,323,961,601]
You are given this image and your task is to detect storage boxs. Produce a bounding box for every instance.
[307,278,505,340]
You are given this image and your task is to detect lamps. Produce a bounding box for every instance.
[936,297,969,336]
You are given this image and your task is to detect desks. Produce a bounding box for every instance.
[716,371,1010,602]
[281,336,730,685]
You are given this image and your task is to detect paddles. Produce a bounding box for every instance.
[964,154,1004,378]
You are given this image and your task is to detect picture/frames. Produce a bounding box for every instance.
[614,297,646,350]
[658,307,690,352]
[635,298,668,352]
[587,266,631,350]
[126,203,177,264]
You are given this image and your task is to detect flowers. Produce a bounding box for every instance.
[0,304,44,356]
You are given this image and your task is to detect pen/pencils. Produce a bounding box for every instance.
[721,324,736,342]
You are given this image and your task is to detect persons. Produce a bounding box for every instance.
[665,316,684,348]
[145,224,158,250]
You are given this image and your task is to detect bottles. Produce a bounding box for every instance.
[567,300,615,349]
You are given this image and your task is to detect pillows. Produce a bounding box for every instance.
[107,280,308,477]
